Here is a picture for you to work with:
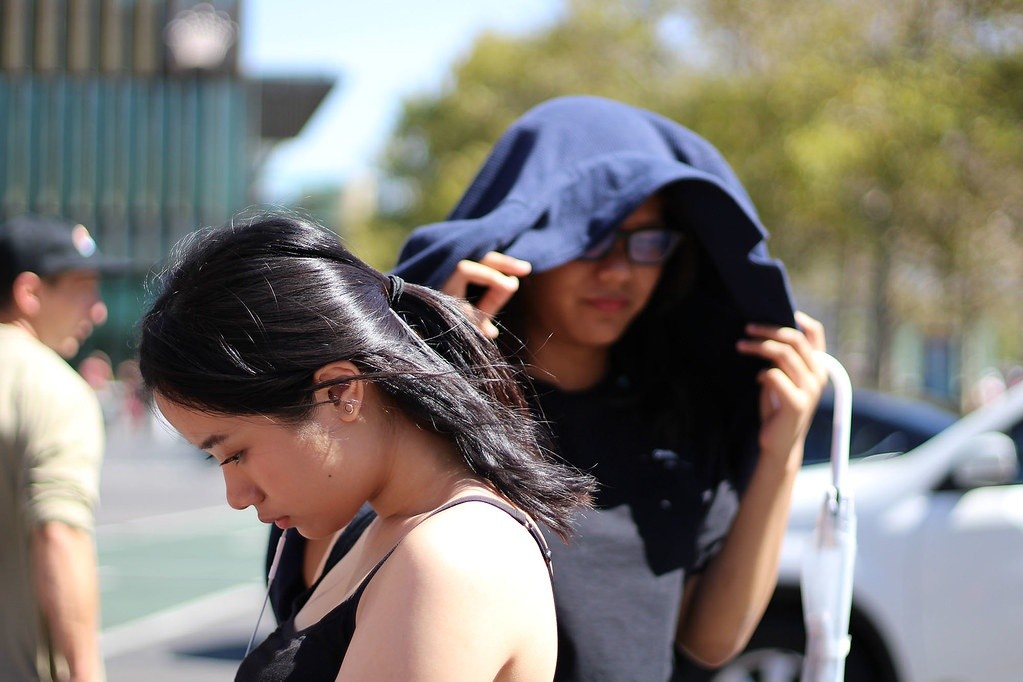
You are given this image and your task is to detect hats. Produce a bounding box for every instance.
[0,216,129,278]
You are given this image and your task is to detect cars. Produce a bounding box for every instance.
[712,378,1023,681]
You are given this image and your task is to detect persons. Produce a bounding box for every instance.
[0,214,133,682]
[139,93,828,682]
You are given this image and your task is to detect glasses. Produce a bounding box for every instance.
[582,224,686,264]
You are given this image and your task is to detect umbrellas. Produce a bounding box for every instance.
[801,354,859,682]
